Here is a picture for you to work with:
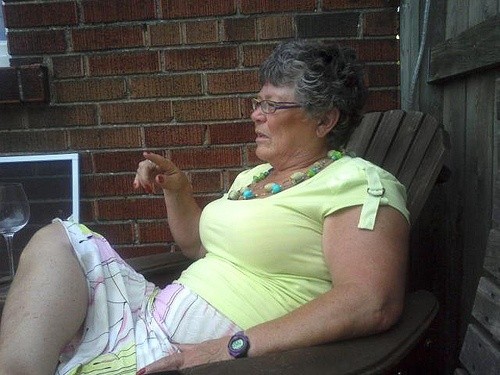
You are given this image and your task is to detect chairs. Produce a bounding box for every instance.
[123,108,491,375]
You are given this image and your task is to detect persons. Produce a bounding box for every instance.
[0,39,410,375]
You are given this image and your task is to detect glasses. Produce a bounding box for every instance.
[250,98,310,114]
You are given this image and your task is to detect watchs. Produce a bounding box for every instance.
[228,330,250,360]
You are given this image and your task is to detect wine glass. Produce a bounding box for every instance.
[0,183,30,294]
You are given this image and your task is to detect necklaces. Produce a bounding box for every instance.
[229,148,350,201]
